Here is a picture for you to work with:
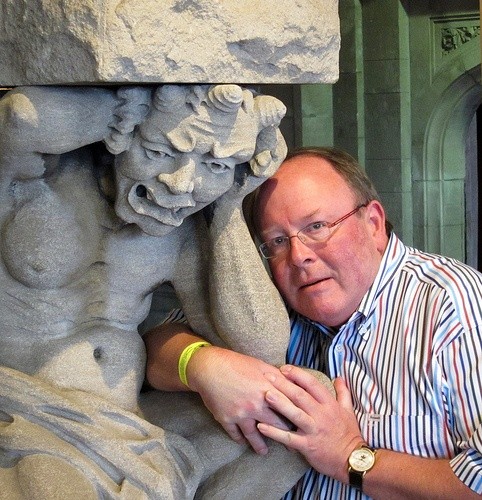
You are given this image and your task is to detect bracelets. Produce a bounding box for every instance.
[177,339,211,397]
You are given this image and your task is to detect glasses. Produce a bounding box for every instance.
[259,203,367,260]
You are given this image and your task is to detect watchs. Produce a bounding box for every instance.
[345,446,380,491]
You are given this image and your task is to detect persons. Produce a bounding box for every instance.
[0,83,309,500]
[143,149,482,500]
